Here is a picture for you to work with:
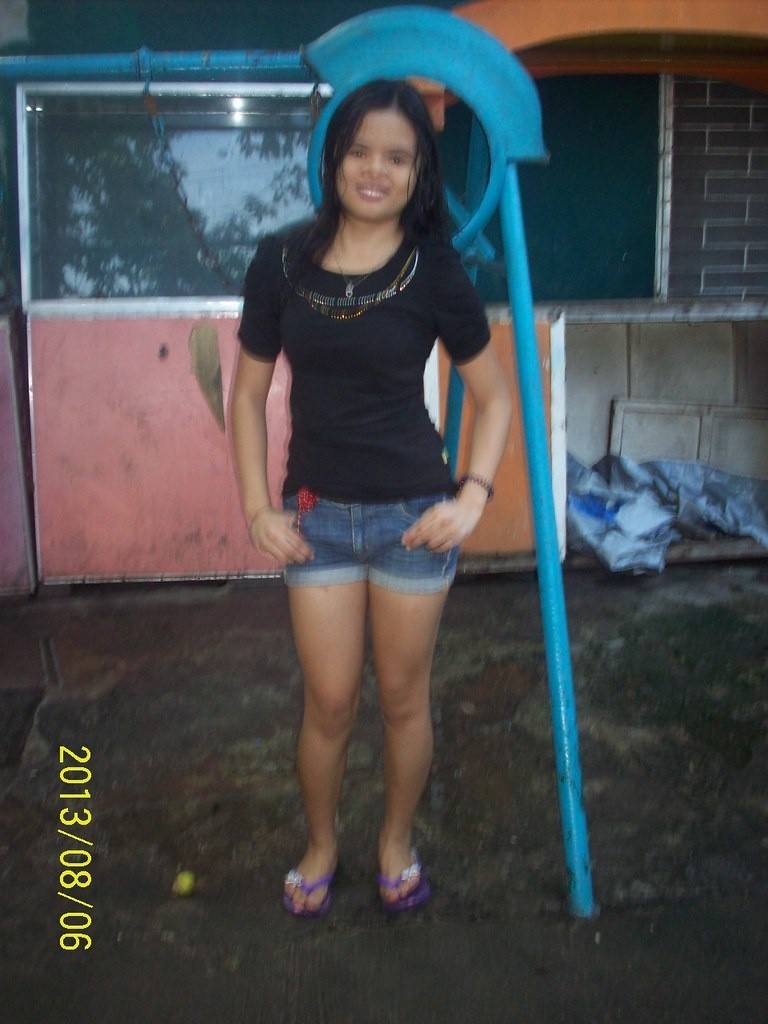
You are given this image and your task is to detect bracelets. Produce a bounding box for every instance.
[456,475,494,503]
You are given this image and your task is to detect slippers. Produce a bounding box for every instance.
[282,867,335,918]
[378,848,430,913]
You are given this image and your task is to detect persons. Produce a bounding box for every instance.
[228,77,513,918]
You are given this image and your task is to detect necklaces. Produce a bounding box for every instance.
[332,244,394,298]
